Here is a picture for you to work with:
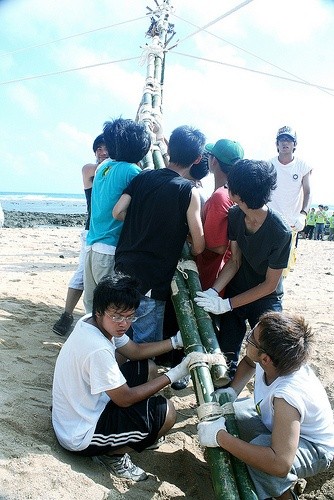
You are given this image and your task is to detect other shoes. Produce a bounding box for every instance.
[170,374,189,391]
[52,312,73,336]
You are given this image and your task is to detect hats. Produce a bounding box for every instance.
[276,126,296,142]
[205,138,244,166]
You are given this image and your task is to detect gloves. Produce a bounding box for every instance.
[194,287,233,315]
[292,213,306,232]
[211,387,238,405]
[163,356,191,384]
[197,416,227,448]
[170,330,184,349]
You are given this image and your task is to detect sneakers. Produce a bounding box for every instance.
[146,435,166,450]
[90,452,148,482]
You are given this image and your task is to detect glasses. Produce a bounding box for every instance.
[104,311,137,322]
[246,330,265,353]
[276,137,293,142]
[224,183,230,190]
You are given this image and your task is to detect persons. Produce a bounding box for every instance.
[300,204,334,241]
[52,117,292,390]
[51,274,191,482]
[196,311,334,500]
[264,125,313,279]
